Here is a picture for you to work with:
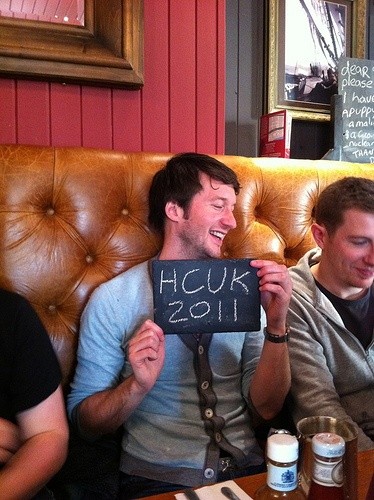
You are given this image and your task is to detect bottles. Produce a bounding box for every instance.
[308,433,345,500]
[252,434,307,500]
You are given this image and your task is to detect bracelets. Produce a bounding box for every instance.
[264,323,291,343]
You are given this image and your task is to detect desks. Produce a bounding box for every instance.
[141,450,374,500]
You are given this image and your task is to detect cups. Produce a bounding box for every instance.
[298,417,359,500]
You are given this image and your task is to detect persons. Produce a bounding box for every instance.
[286,176,374,454]
[65,151,292,500]
[0,288,70,500]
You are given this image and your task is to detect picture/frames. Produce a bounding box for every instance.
[266,0,368,122]
[0,0,145,90]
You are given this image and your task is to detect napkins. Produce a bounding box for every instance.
[174,480,253,500]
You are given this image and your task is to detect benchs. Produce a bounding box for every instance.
[0,143,374,500]
